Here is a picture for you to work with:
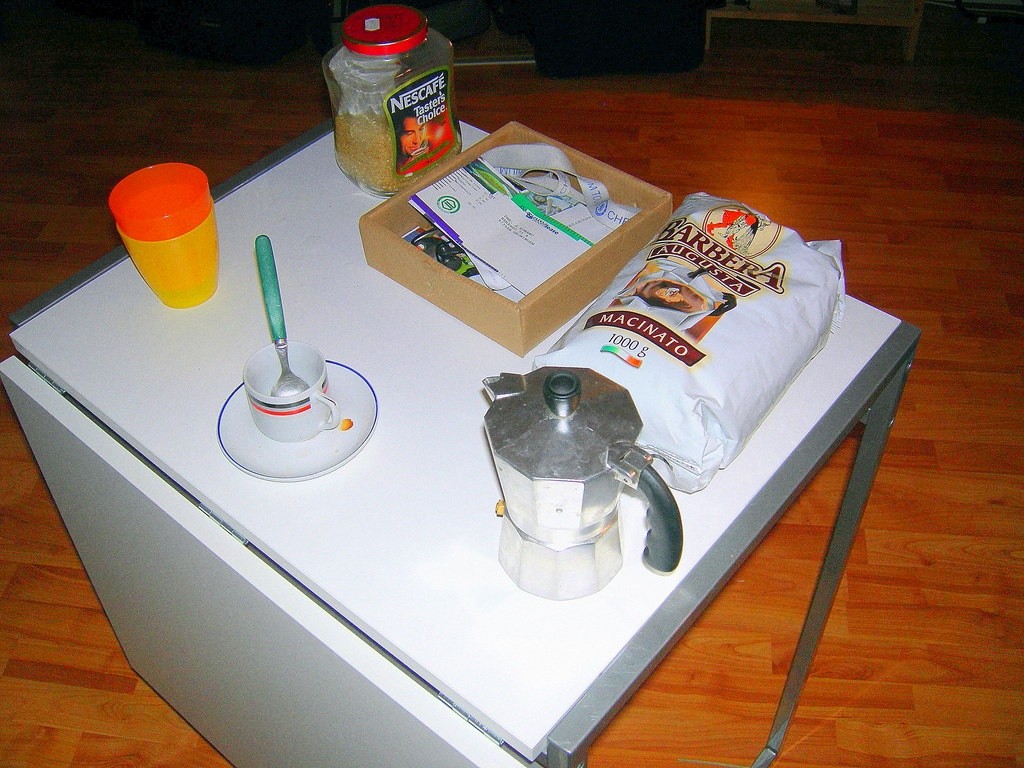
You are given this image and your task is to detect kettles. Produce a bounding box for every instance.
[482,366,683,600]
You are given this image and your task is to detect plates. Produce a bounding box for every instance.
[217,360,377,482]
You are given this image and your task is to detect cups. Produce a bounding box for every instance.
[244,340,340,442]
[106,162,218,309]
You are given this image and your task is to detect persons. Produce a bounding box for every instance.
[395,110,425,164]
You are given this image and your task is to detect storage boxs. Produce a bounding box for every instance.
[359,119,675,357]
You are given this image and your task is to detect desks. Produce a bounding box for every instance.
[0,116,924,768]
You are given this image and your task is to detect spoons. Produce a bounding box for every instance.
[255,235,309,396]
